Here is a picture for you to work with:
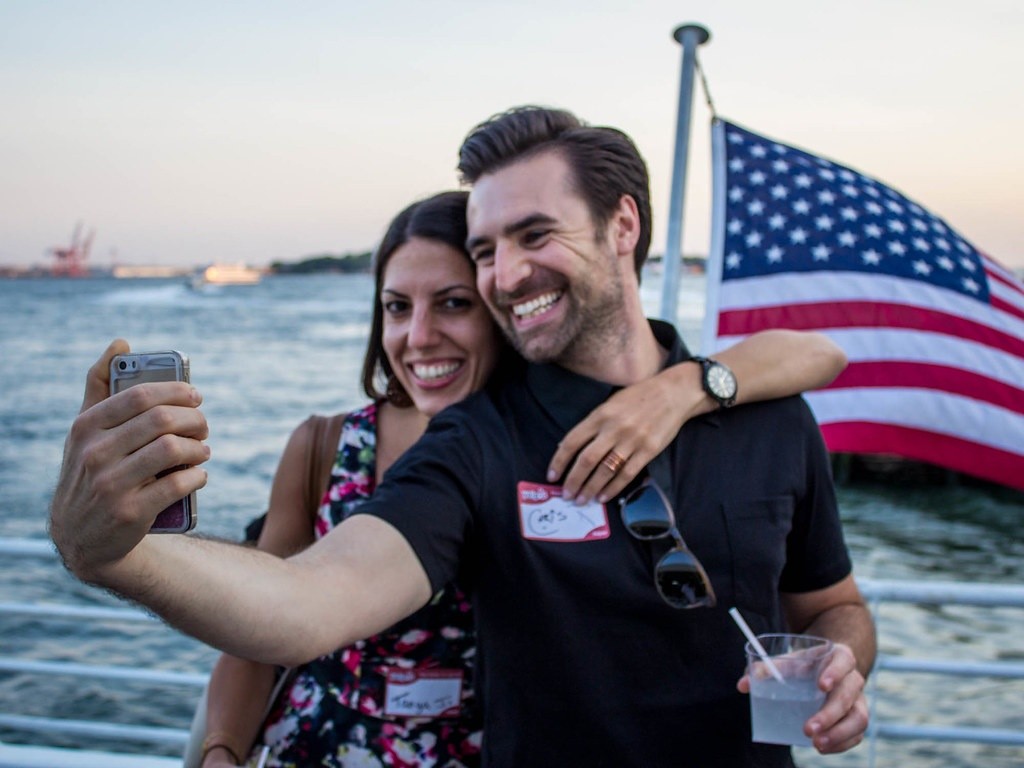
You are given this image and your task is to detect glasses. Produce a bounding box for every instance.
[617,476,717,610]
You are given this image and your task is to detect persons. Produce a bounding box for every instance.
[174,189,845,768]
[48,106,878,768]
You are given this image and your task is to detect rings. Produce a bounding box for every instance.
[604,451,625,473]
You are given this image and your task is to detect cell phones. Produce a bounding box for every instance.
[109,349,197,535]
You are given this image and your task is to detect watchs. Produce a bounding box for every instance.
[682,352,738,408]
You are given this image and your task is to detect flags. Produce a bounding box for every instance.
[706,115,1024,492]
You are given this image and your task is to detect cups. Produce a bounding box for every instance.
[744,632,836,748]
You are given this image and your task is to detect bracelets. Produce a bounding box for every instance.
[200,744,241,765]
